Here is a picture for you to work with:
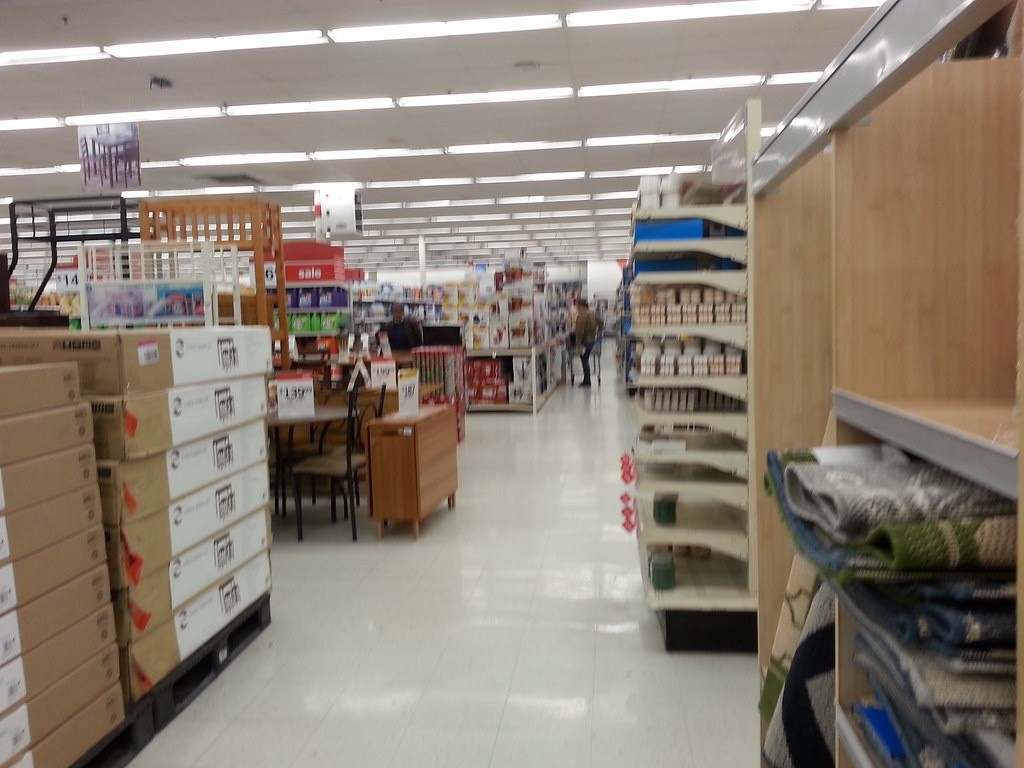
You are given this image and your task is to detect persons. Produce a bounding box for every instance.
[571,298,604,386]
[374,303,422,349]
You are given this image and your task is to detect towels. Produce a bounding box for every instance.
[756,440,1016,768]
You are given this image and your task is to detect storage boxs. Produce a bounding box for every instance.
[468,293,542,403]
[1,324,275,768]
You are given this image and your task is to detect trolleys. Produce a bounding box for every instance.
[565,333,603,388]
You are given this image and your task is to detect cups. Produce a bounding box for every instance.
[640,173,682,208]
[648,545,675,589]
[652,489,677,524]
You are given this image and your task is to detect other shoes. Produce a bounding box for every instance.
[578,382,591,388]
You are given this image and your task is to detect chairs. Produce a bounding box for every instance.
[269,376,389,542]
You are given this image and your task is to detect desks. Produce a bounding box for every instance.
[266,403,361,518]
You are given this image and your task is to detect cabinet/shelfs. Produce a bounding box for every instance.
[615,0,1024,768]
[363,404,458,540]
[263,279,351,363]
[350,293,445,329]
[467,330,577,415]
[137,194,291,371]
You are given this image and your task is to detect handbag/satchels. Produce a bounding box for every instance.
[569,332,576,342]
